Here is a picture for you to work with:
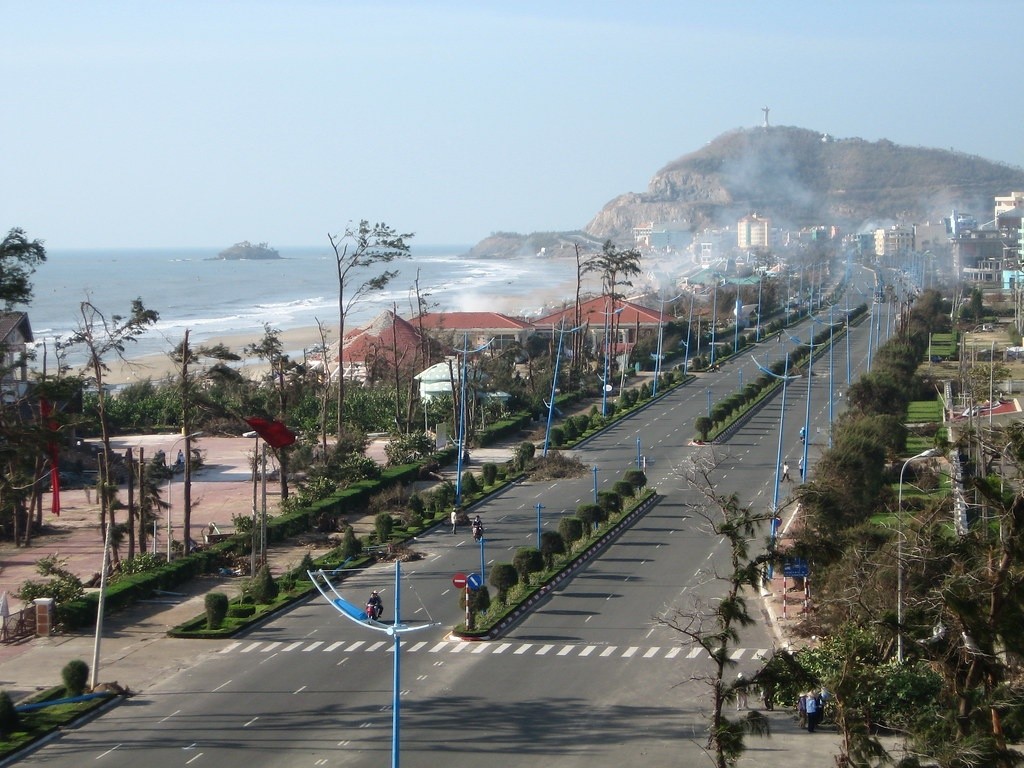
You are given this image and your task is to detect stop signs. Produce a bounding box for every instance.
[453,574,467,588]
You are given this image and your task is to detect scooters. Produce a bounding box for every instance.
[362,602,381,624]
[472,522,483,542]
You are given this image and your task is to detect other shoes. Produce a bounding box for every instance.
[378,614,383,619]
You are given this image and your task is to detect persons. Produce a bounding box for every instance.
[759,692,772,711]
[798,456,806,478]
[472,516,483,541]
[366,590,384,620]
[780,461,790,482]
[737,672,750,711]
[799,687,831,733]
[800,427,806,444]
[451,508,458,534]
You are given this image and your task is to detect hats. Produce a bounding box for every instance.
[738,672,743,679]
[372,589,379,594]
[798,692,806,697]
[808,691,815,699]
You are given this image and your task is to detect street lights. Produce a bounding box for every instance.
[599,302,632,417]
[305,558,444,768]
[749,354,804,583]
[452,331,496,506]
[543,316,589,457]
[651,292,686,398]
[780,328,827,486]
[895,449,938,663]
[167,430,203,561]
[987,340,998,427]
[998,440,1013,541]
[807,298,892,446]
